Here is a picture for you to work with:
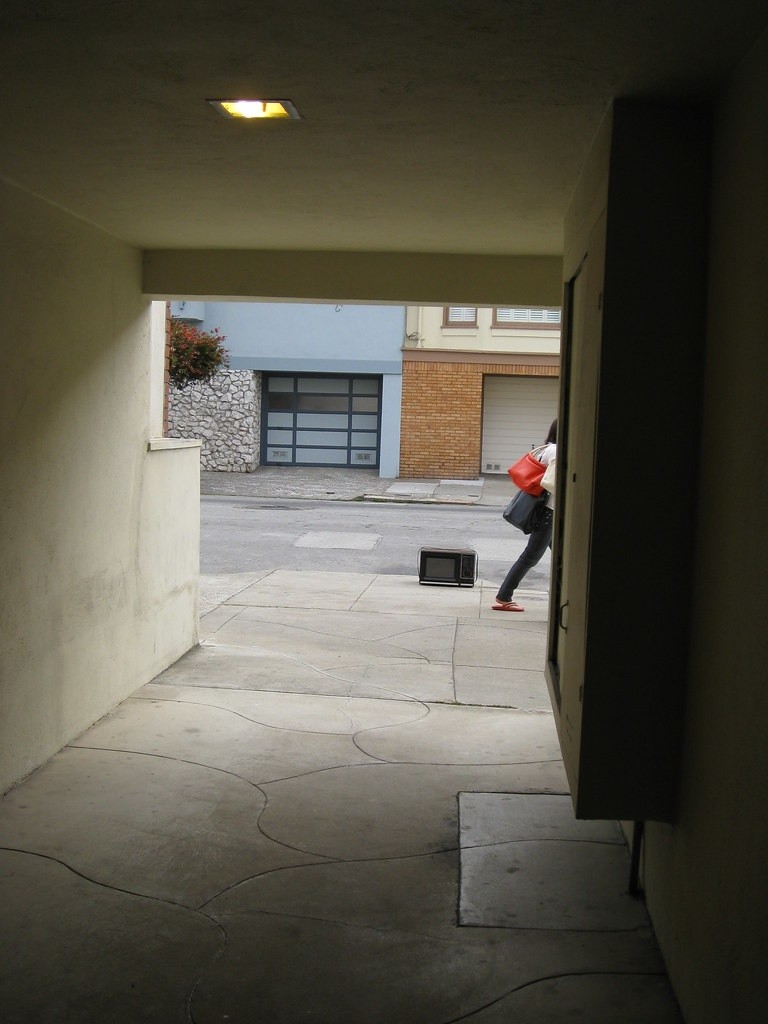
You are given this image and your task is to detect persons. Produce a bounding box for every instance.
[492,418,557,612]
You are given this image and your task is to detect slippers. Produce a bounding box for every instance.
[492,601,524,611]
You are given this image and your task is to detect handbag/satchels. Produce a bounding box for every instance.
[503,488,549,535]
[508,444,550,496]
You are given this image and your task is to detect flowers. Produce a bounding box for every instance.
[168,314,232,392]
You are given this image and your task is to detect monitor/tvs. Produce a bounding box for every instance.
[419,548,476,587]
